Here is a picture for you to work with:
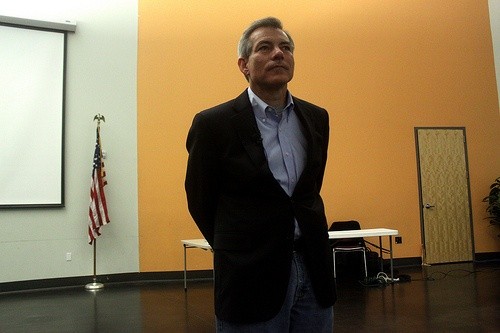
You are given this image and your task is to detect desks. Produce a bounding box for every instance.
[181,227,401,292]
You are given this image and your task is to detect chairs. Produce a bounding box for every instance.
[328,222,368,278]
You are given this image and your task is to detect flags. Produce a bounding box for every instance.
[88,114,111,245]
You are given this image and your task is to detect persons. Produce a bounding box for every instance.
[185,16,337,333]
